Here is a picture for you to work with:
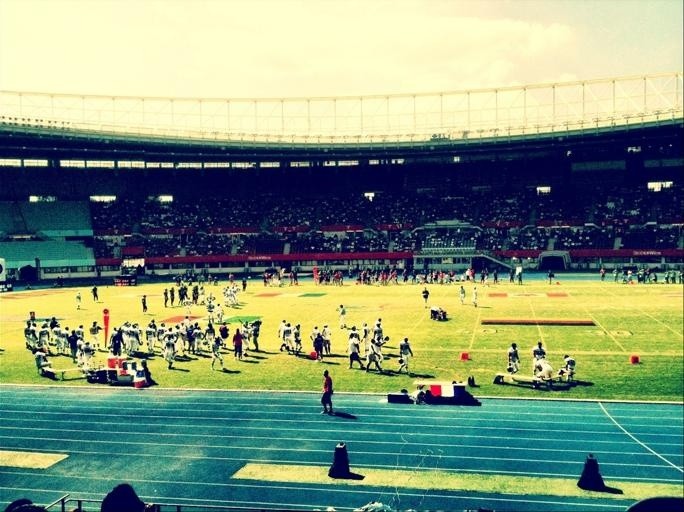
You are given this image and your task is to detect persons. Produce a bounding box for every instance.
[5,483,146,512]
[319,369,334,416]
